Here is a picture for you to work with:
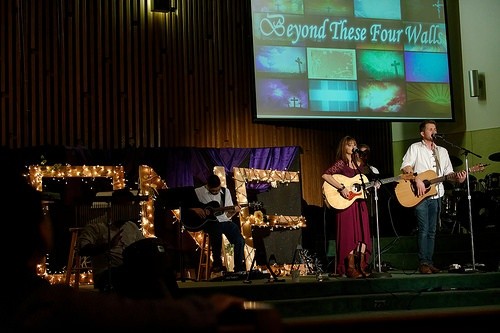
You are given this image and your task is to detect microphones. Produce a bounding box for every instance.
[354,148,367,155]
[433,133,443,138]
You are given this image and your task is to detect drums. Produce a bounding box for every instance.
[444,171,500,232]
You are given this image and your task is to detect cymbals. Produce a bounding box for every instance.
[450,156,463,167]
[488,152,500,162]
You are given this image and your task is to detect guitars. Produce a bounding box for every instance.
[181,200,264,232]
[321,171,418,212]
[392,162,485,209]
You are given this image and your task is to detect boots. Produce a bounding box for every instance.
[358,252,370,277]
[347,254,363,279]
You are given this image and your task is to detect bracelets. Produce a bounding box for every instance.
[337,186,345,192]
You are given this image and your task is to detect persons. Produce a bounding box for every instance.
[76,189,148,294]
[321,135,382,278]
[190,174,246,278]
[400,119,467,274]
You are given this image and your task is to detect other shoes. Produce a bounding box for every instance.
[420,267,432,274]
[431,265,440,274]
